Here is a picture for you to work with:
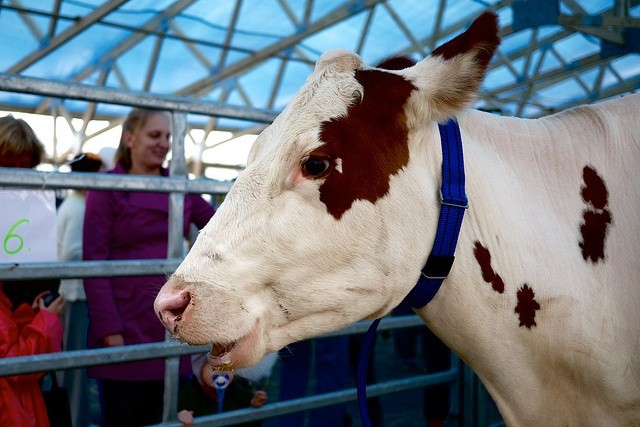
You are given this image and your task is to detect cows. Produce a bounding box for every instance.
[152,7,640,427]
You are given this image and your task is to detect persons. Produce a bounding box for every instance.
[82,107,217,426]
[0,287,65,427]
[0,116,58,312]
[176,347,266,427]
[59,151,106,427]
